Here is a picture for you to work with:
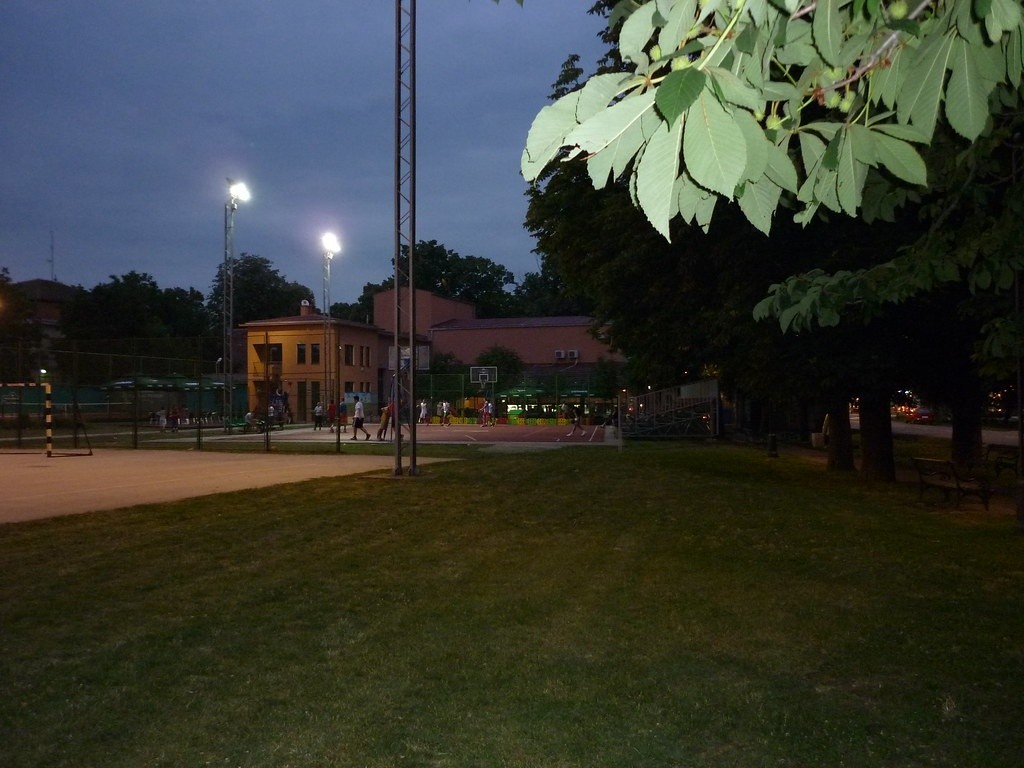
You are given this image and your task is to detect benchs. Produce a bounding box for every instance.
[912,441,1024,513]
[240,414,285,432]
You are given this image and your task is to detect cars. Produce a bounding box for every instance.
[905,407,936,425]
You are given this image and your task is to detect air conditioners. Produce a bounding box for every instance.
[554,349,565,359]
[568,349,578,359]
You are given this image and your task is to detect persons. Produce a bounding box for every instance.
[351,396,371,441]
[337,396,347,433]
[417,399,428,423]
[149,403,290,433]
[313,402,323,431]
[377,401,394,440]
[487,400,495,427]
[823,412,831,448]
[478,398,490,428]
[326,398,336,433]
[600,407,617,429]
[565,403,588,438]
[435,399,451,427]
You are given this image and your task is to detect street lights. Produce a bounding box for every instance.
[222,179,250,436]
[321,230,342,425]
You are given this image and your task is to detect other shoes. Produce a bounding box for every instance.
[481,424,493,427]
[351,437,357,440]
[447,423,452,426]
[365,433,371,440]
[440,423,444,426]
